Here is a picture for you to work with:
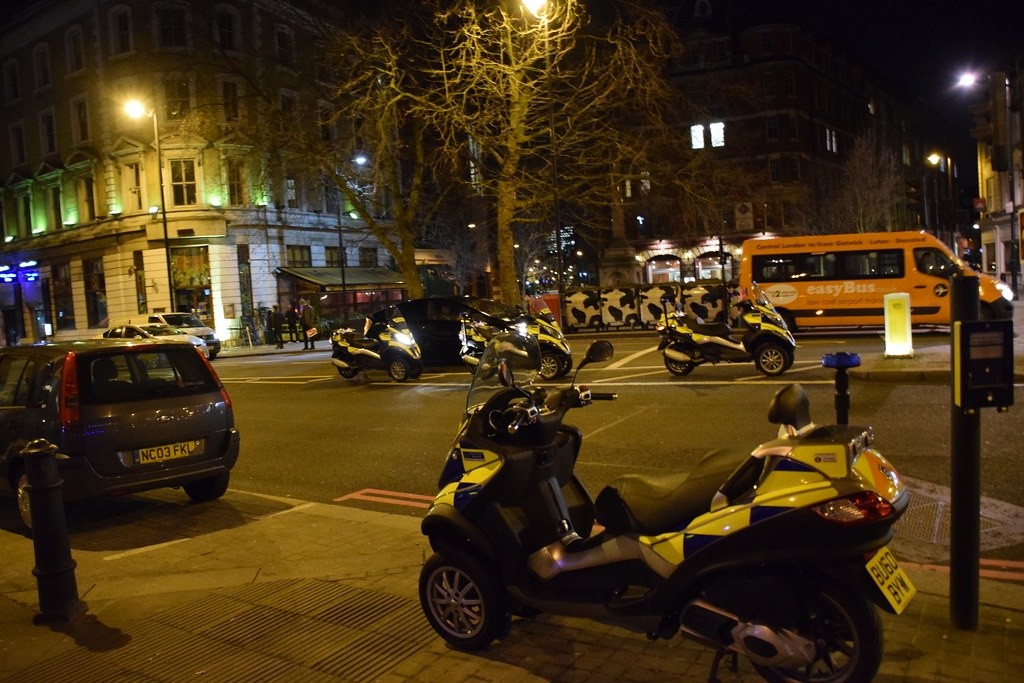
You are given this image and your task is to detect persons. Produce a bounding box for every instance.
[919,250,936,275]
[297,298,317,351]
[964,235,984,272]
[265,310,276,345]
[285,305,302,343]
[524,274,554,296]
[272,304,284,349]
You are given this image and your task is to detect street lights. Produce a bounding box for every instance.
[122,100,175,314]
[334,155,367,325]
[959,71,1020,301]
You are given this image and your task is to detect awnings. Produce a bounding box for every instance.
[278,265,408,292]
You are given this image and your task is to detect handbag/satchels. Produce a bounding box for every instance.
[306,325,318,339]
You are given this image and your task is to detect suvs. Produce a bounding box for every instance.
[1,336,240,535]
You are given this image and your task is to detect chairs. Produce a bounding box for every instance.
[90,359,130,402]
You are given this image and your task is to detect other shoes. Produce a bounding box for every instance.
[310,347,314,350]
[303,347,308,350]
[275,345,280,349]
[280,345,283,349]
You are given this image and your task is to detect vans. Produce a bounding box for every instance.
[108,312,222,360]
[739,229,1015,336]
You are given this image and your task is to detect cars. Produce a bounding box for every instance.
[345,296,518,367]
[92,323,209,360]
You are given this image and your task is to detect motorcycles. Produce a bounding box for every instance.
[656,282,797,376]
[330,306,421,382]
[458,296,574,381]
[416,329,917,682]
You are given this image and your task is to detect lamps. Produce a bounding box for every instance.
[33,228,44,237]
[64,220,76,227]
[257,201,268,207]
[110,210,123,217]
[149,204,160,215]
[5,235,15,242]
[211,201,222,208]
